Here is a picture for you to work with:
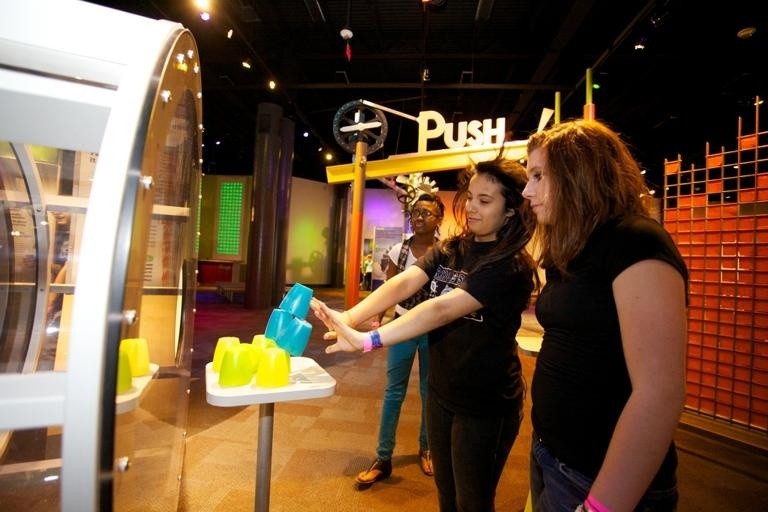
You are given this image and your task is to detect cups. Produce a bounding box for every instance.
[218,348,253,388]
[275,318,314,357]
[240,333,292,388]
[279,283,314,319]
[264,309,294,340]
[213,337,241,374]
[113,351,134,396]
[117,338,151,376]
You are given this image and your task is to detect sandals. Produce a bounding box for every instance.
[356,458,392,485]
[419,448,435,477]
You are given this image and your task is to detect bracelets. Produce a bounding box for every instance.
[583,496,614,512]
[363,335,371,356]
[369,330,384,351]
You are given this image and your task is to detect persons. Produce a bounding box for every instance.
[308,157,542,511]
[354,193,445,489]
[520,116,688,512]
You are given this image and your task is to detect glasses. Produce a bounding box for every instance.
[410,209,439,220]
